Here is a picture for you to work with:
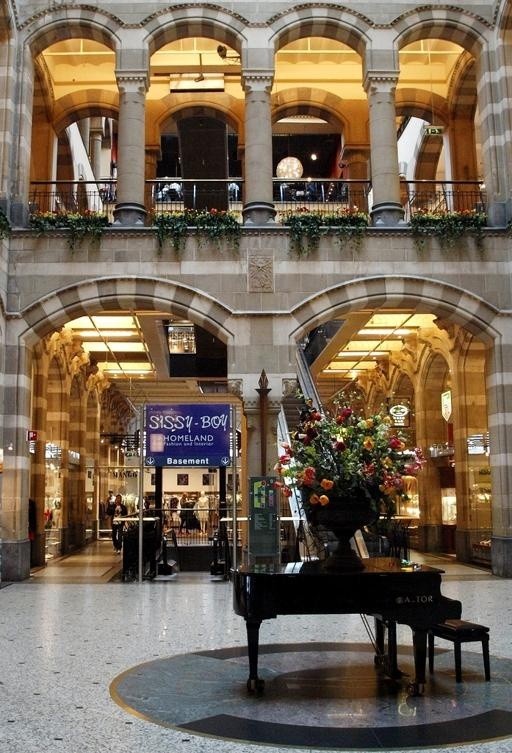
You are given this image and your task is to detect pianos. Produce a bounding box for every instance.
[231,557,462,696]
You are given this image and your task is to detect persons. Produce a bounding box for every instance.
[107,493,129,554]
[105,489,117,536]
[158,174,318,202]
[197,488,210,533]
[298,396,317,424]
[176,493,192,536]
[144,496,150,516]
[142,497,146,516]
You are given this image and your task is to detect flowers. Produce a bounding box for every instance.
[272,386,427,522]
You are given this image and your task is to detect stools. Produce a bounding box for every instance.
[427,619,490,682]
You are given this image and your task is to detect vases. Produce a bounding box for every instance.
[316,505,373,570]
